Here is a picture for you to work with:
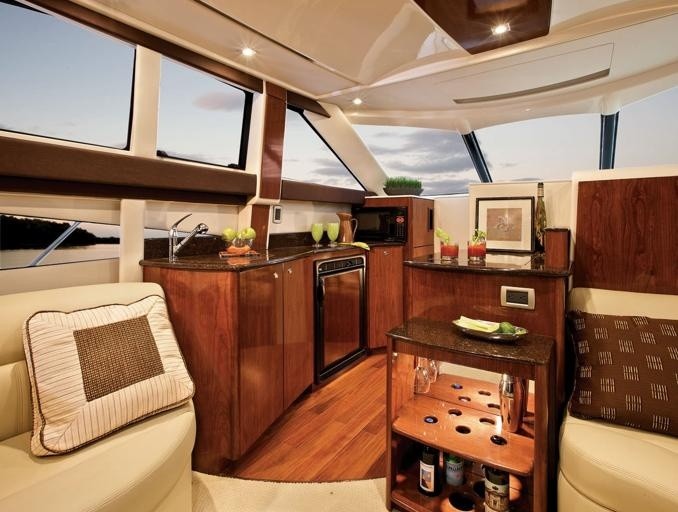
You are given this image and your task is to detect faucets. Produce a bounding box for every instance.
[168,213,209,262]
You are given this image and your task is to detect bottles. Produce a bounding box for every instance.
[533,181,547,255]
[497,374,527,435]
[415,444,510,512]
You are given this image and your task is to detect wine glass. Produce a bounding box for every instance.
[409,355,439,396]
[310,222,324,249]
[327,221,340,248]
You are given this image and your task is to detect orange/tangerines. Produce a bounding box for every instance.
[500,321,515,334]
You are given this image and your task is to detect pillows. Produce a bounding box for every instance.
[560,301,678,442]
[15,293,198,462]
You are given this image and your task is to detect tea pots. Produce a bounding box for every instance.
[335,211,359,243]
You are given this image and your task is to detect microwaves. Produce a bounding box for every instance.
[351,206,407,244]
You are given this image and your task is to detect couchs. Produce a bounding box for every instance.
[554,284,678,512]
[1,278,196,512]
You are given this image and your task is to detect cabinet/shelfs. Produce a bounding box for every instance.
[136,250,310,481]
[408,196,435,249]
[374,300,560,511]
[407,244,434,261]
[365,244,410,357]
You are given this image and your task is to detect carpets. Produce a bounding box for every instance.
[185,466,390,511]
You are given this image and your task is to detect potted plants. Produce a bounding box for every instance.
[380,175,425,196]
[433,223,459,262]
[466,226,488,263]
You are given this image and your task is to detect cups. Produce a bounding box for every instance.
[440,239,459,261]
[467,240,486,262]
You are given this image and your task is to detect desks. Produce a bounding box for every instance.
[396,243,574,432]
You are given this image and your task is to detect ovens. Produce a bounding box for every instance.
[312,254,369,385]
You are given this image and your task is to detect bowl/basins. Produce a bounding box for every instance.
[224,236,254,254]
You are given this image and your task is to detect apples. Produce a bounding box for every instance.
[222,227,256,240]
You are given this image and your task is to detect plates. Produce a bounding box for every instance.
[544,227,569,232]
[451,319,527,343]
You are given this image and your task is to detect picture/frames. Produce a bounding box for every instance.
[473,193,538,255]
